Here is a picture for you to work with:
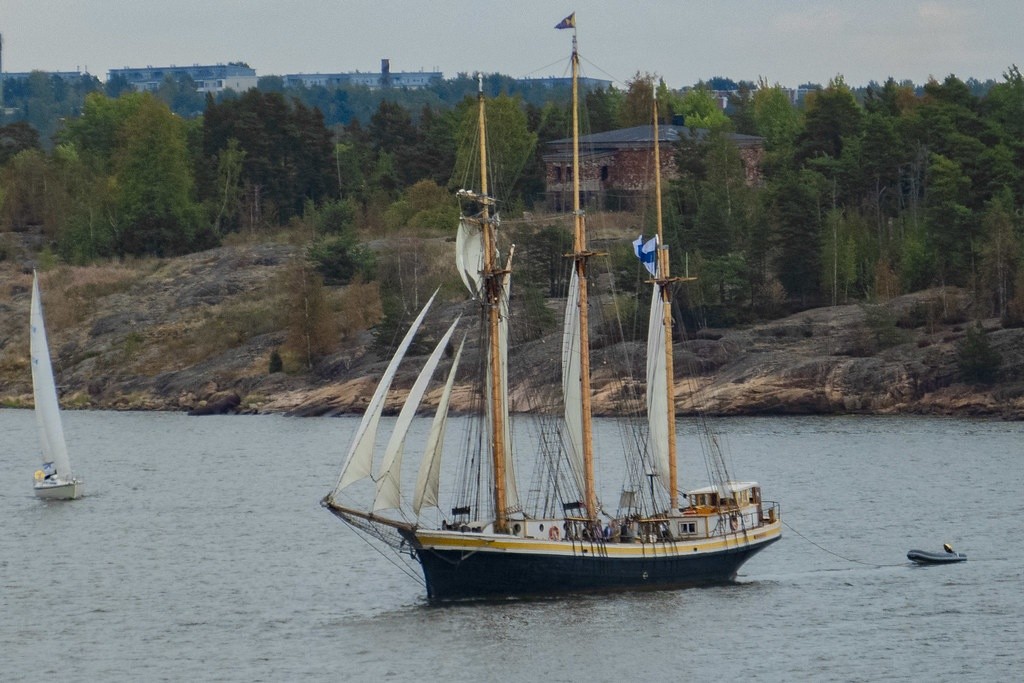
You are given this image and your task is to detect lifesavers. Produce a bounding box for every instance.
[549,526,560,540]
[730,517,738,530]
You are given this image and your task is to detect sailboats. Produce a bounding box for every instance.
[28,265,85,500]
[315,11,785,609]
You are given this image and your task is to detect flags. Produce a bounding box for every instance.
[632,236,658,277]
